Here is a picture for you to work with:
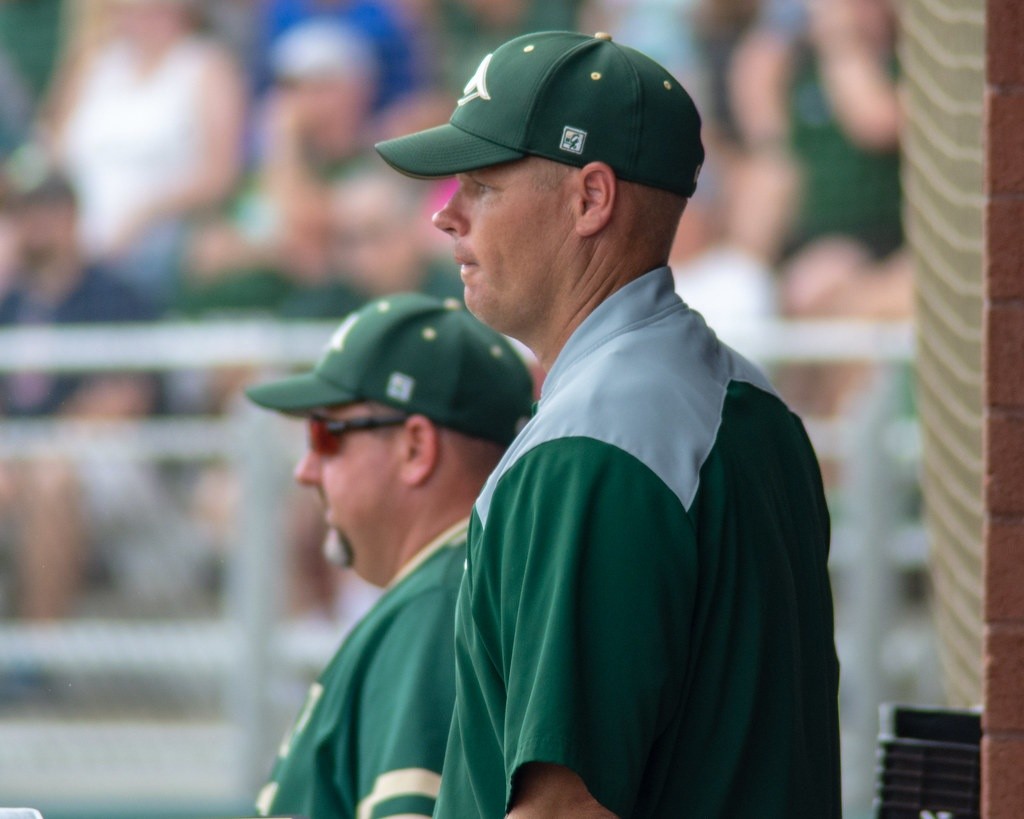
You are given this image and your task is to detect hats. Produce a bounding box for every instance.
[374,32,703,199]
[244,288,532,448]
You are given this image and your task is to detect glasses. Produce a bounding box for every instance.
[309,414,415,456]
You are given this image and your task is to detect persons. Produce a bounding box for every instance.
[1,0,928,701]
[373,31,844,819]
[244,294,534,819]
